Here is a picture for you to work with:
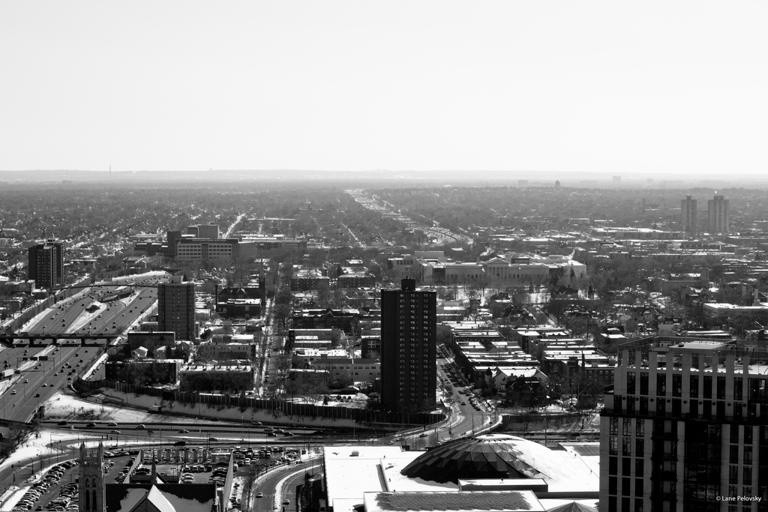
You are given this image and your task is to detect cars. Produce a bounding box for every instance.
[543,394,604,412]
[10,275,165,398]
[436,342,504,412]
[262,335,352,403]
[12,420,301,511]
[418,432,428,438]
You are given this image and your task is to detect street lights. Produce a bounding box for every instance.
[241,415,244,444]
